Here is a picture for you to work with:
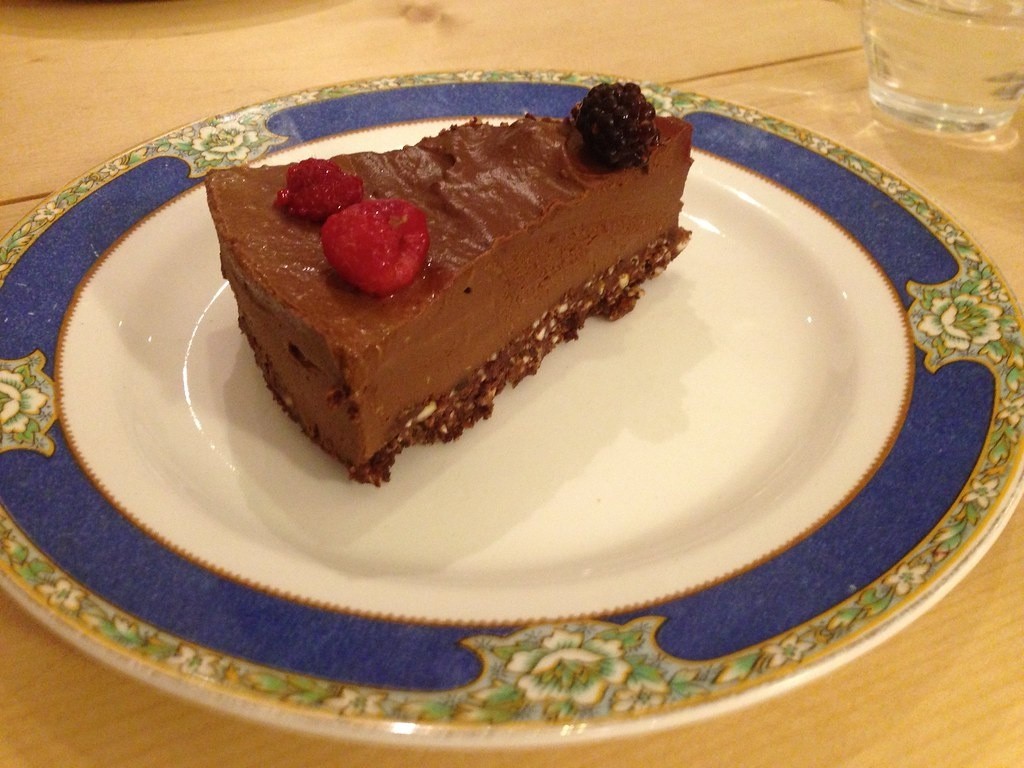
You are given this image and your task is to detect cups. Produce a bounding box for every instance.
[860,0,1024,139]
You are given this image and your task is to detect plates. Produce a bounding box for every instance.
[0,67,1024,751]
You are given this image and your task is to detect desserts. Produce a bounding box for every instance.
[201,81,695,487]
